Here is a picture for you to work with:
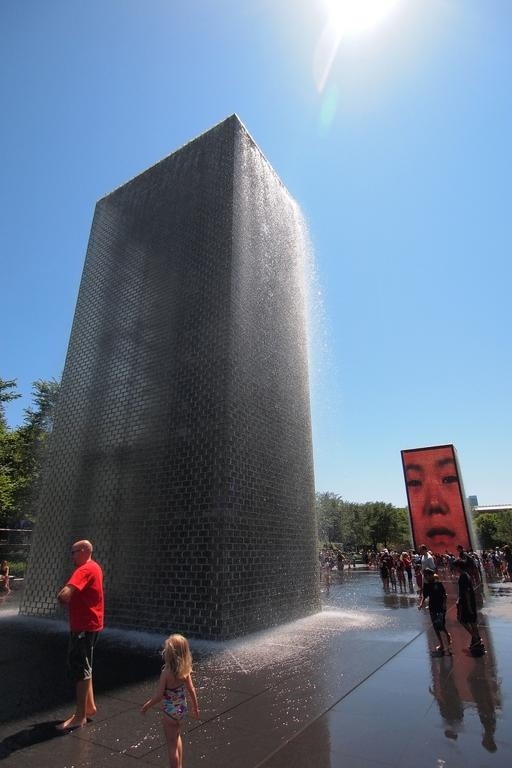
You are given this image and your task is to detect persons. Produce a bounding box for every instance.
[319,544,512,594]
[401,447,471,558]
[141,634,199,768]
[452,559,484,649]
[0,560,11,596]
[418,568,452,650]
[55,539,105,730]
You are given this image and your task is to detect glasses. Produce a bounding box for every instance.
[71,549,81,556]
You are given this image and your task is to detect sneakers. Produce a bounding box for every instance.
[470,637,483,648]
